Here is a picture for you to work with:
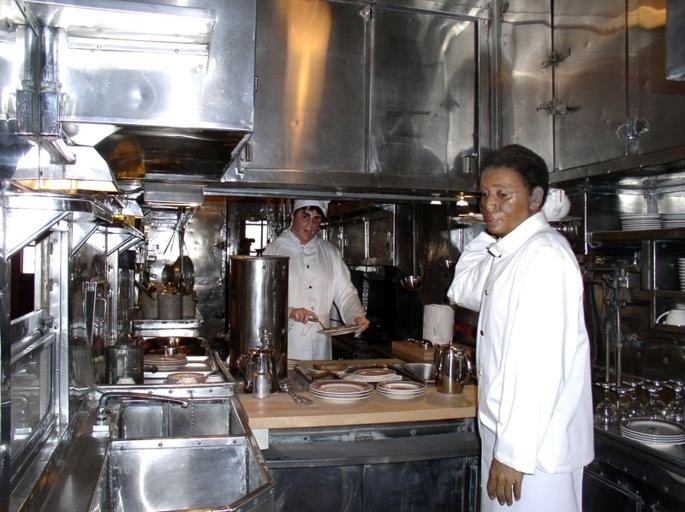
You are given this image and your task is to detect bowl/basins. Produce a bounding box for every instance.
[664,309,684,326]
[400,275,424,290]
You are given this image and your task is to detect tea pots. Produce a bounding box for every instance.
[541,187,571,221]
[434,343,472,395]
[237,346,287,394]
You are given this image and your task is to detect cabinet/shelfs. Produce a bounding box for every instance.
[590,227,685,335]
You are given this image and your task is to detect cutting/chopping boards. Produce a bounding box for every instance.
[293,357,406,383]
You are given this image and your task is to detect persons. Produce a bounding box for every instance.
[262,199,371,361]
[446,144,596,512]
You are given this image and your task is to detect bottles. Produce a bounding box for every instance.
[251,373,270,398]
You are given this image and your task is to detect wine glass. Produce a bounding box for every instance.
[595,379,685,432]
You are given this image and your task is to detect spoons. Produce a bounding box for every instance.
[279,383,302,404]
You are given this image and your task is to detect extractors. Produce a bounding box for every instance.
[8,25,119,197]
[114,199,144,220]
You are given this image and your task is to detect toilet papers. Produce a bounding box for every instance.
[422,304,455,345]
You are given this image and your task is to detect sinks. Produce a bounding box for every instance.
[143,371,225,385]
[111,396,246,438]
[89,436,276,512]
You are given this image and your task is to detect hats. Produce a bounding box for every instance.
[293,199,331,217]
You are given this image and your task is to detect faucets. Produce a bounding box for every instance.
[584,260,622,388]
[100,393,189,408]
[144,365,158,375]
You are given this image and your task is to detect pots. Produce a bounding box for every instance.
[169,228,195,296]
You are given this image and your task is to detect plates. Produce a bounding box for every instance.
[375,381,425,401]
[143,353,188,371]
[342,369,403,382]
[309,379,375,404]
[318,325,362,337]
[619,417,685,447]
[618,213,662,231]
[660,212,685,230]
[167,372,205,385]
[678,257,685,294]
